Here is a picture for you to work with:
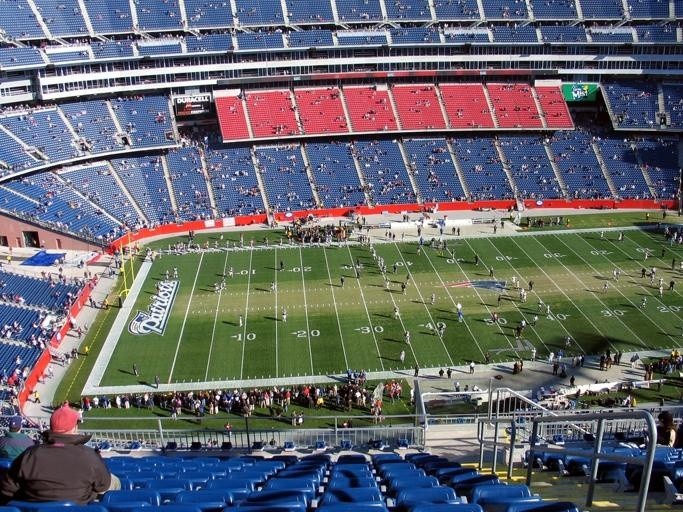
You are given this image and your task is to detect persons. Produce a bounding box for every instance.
[0,0,682,249]
[0,406,121,505]
[0,221,682,463]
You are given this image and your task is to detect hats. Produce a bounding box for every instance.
[50,407,81,433]
[9,416,21,428]
